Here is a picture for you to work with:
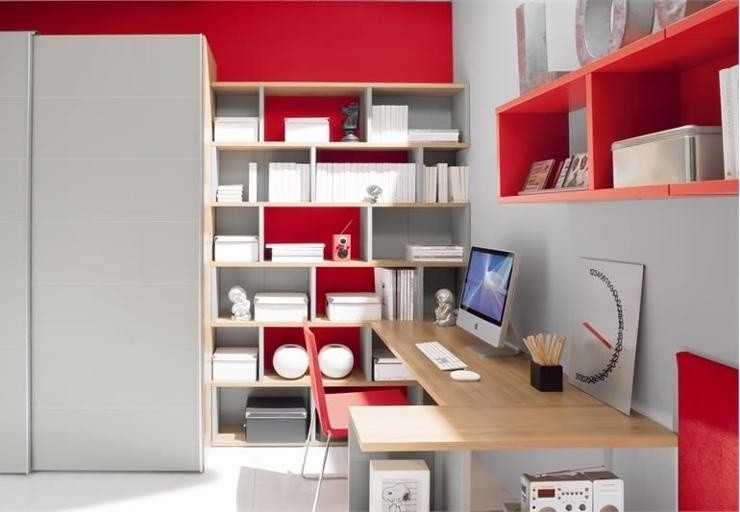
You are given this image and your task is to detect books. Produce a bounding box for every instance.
[370,105,460,142]
[266,242,326,262]
[216,160,467,204]
[373,243,463,321]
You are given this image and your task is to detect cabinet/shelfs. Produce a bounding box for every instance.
[496,1,739,203]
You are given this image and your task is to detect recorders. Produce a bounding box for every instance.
[520,471,625,512]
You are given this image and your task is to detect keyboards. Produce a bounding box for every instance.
[414,341,469,371]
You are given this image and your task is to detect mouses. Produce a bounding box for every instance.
[450,370,481,382]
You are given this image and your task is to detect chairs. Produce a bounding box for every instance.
[295,321,411,511]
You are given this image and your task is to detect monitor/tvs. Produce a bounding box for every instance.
[455,244,521,358]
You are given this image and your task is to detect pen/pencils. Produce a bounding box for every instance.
[522,332,566,366]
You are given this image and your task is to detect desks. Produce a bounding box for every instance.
[347,318,680,511]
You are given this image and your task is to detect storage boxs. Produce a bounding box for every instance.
[281,115,329,146]
[252,290,308,324]
[243,395,308,443]
[322,291,384,323]
[211,347,258,382]
[609,123,726,190]
[212,117,258,146]
[212,232,256,264]
